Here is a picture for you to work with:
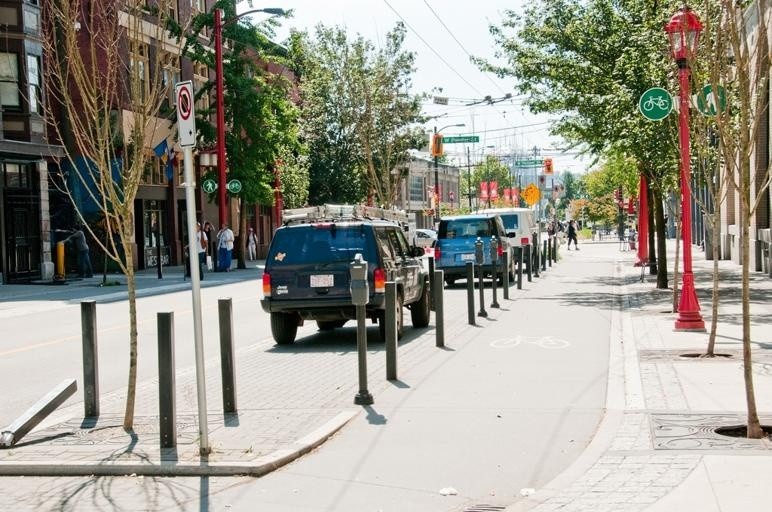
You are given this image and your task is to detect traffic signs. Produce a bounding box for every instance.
[441,135,479,143]
[515,160,545,166]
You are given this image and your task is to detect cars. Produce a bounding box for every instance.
[415,225,439,250]
[546,220,609,238]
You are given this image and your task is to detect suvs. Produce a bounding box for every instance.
[433,214,516,289]
[259,200,431,346]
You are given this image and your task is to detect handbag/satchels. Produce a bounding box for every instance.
[201,239,206,248]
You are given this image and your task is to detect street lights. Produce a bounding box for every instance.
[664,1,710,333]
[215,6,287,228]
[433,122,468,220]
[468,145,496,212]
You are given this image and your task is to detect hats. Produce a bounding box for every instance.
[248,228,253,231]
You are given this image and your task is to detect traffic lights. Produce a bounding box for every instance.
[545,159,553,174]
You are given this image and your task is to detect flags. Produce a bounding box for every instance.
[152,138,174,182]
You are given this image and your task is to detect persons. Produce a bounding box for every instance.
[627,225,639,250]
[184,220,259,281]
[547,219,580,251]
[55,224,94,278]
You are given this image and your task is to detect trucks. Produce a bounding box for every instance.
[475,206,545,265]
